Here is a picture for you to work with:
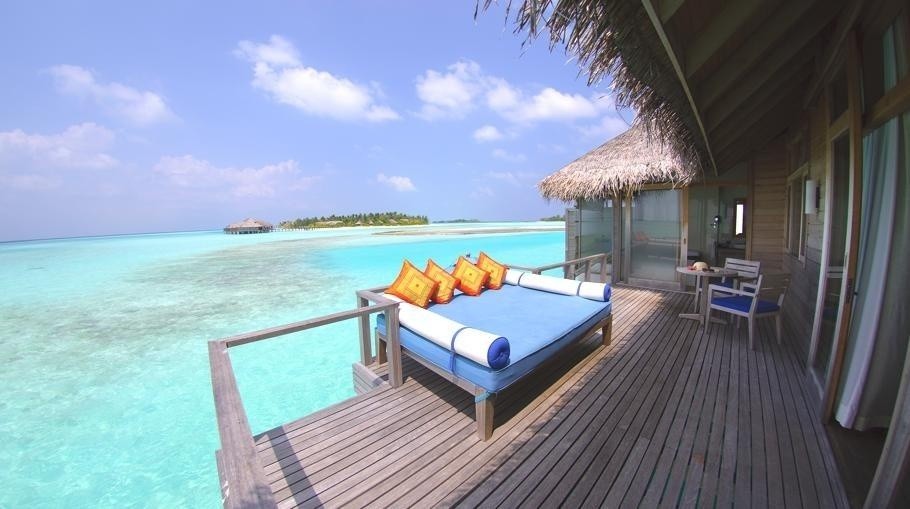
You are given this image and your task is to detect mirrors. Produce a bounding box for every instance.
[732,198,746,240]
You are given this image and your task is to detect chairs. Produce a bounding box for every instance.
[702,257,793,352]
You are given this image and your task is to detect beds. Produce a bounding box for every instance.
[376,268,612,440]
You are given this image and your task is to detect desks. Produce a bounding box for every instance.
[676,266,738,325]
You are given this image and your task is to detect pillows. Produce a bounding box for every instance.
[383,251,508,310]
